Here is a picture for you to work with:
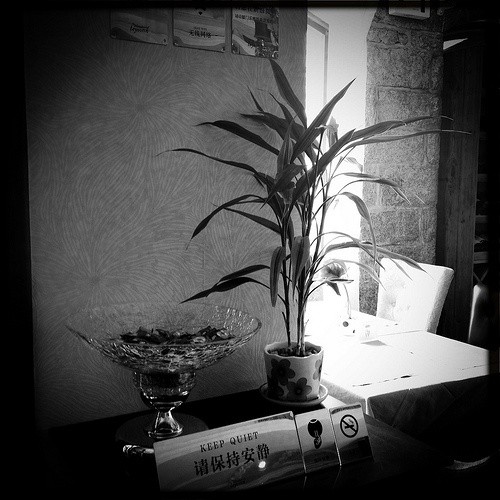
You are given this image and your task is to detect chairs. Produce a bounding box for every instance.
[375,257,455,334]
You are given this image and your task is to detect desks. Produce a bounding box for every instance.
[0,300,500,500]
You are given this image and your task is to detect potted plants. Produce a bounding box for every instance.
[153,57,473,407]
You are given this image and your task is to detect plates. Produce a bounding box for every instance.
[66,302,262,438]
